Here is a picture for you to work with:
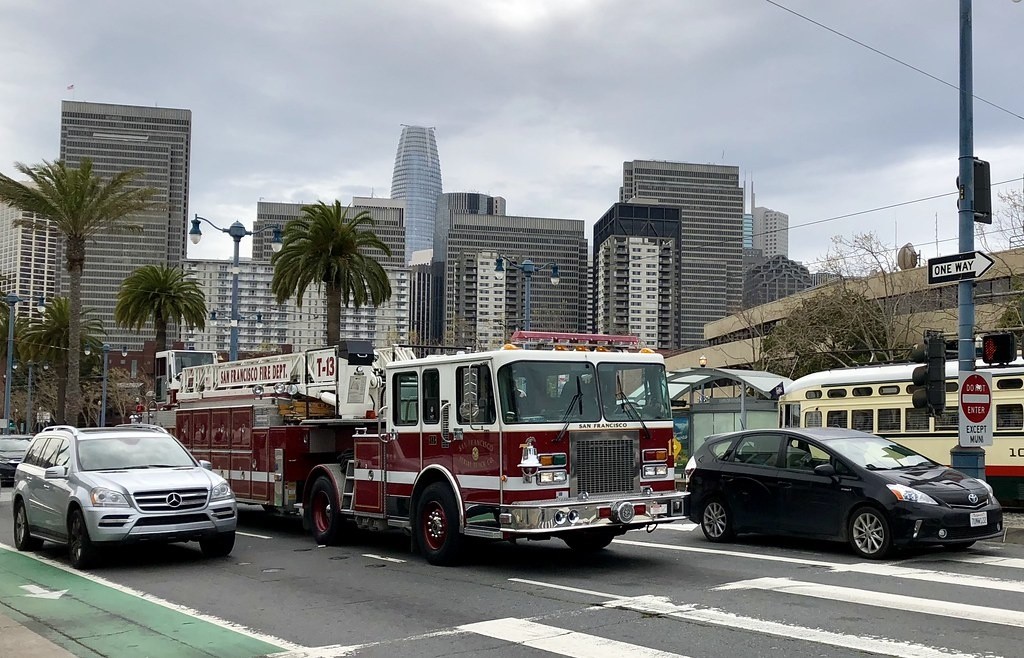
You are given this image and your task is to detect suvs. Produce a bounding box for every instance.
[11,423,239,570]
[680,426,1004,560]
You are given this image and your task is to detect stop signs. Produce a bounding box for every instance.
[959,372,995,424]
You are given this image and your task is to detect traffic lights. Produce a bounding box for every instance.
[983,333,1015,368]
[910,337,947,413]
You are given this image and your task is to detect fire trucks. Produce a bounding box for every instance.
[146,332,694,570]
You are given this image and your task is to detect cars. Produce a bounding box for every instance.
[0,434,35,486]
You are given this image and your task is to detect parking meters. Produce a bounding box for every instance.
[85,344,128,426]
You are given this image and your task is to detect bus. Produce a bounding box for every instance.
[776,350,1024,520]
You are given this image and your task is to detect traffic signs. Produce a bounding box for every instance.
[926,251,997,285]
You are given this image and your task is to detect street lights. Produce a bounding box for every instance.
[0,293,46,435]
[12,357,49,433]
[208,310,265,362]
[493,249,561,332]
[188,214,283,363]
